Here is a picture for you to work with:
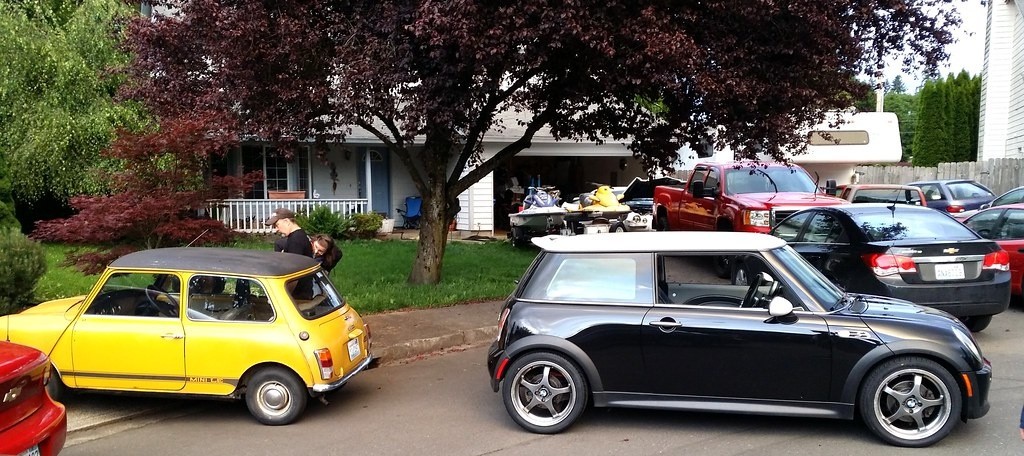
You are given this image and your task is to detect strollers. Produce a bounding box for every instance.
[396,196,423,229]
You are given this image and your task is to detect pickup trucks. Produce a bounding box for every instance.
[653,162,850,234]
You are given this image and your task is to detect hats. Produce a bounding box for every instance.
[265,208,294,226]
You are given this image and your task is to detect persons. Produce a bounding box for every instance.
[265,208,316,302]
[308,233,343,274]
[273,231,289,253]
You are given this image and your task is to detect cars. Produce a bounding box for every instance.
[953,203,1024,297]
[730,203,1011,333]
[906,179,998,214]
[822,184,926,207]
[1,342,68,456]
[950,186,1024,218]
[488,235,993,448]
[0,247,374,427]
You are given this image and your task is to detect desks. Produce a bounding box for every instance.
[268,191,306,199]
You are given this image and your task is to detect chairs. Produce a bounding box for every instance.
[217,279,254,320]
[396,196,423,228]
